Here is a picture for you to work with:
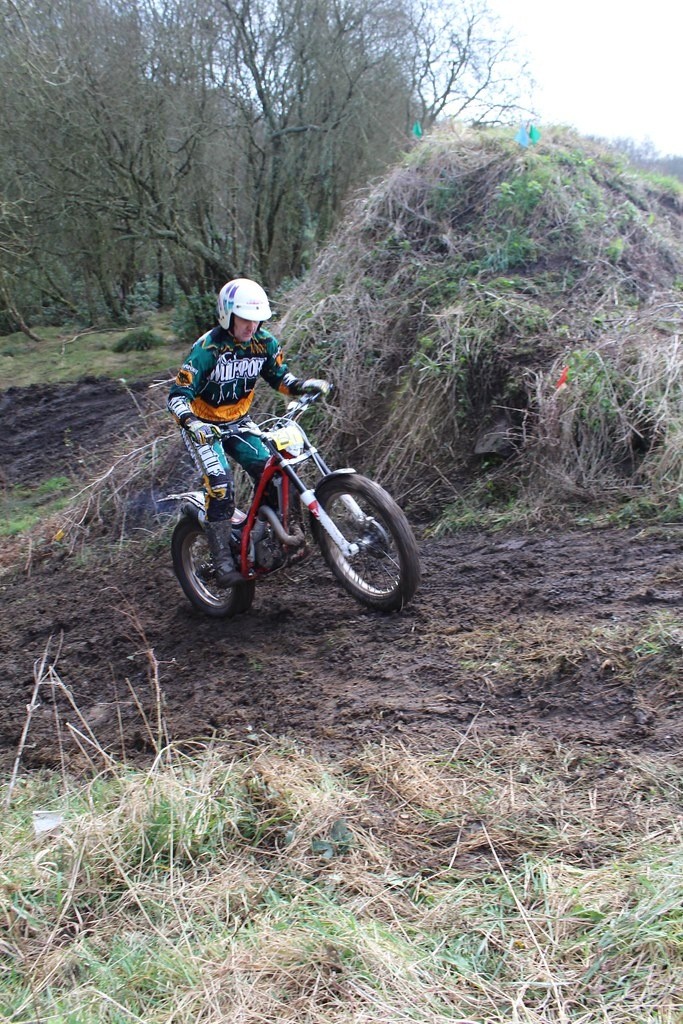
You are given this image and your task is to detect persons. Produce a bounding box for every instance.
[166,275,333,589]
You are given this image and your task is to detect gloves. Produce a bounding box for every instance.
[295,377,332,396]
[183,416,222,445]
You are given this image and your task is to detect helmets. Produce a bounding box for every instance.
[216,278,271,330]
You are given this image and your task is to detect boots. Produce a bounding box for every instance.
[204,518,242,589]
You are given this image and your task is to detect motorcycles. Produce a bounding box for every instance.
[155,381,420,620]
